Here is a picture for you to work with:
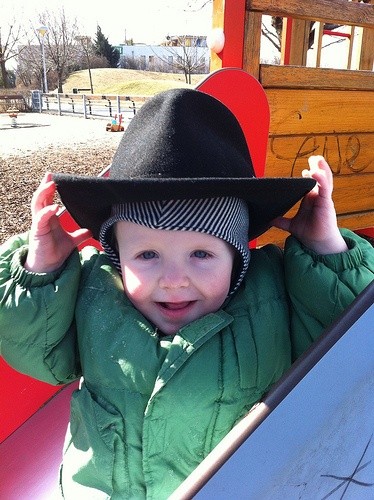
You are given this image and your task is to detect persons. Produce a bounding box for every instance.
[0,87,373,500]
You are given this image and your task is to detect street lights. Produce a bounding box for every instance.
[36,26,50,110]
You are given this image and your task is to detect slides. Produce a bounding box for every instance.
[0,65,373,500]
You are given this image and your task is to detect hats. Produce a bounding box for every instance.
[52,88,316,244]
